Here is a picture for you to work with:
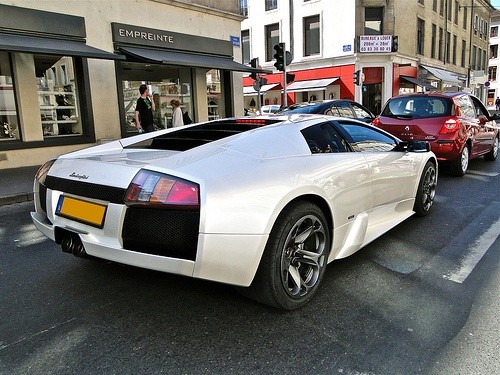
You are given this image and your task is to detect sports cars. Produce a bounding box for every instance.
[30,113,439,311]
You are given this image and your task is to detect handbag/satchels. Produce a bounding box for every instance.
[182,111,193,125]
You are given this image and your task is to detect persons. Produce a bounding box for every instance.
[495,97,500,111]
[170,99,184,127]
[273,97,278,104]
[265,98,269,105]
[135,84,155,134]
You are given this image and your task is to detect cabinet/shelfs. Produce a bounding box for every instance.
[0,85,17,141]
[207,95,220,118]
[124,94,190,119]
[38,91,82,138]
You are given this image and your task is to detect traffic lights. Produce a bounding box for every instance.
[353,71,359,85]
[273,43,284,71]
[248,58,256,80]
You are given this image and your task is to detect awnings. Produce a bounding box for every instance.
[0,32,126,60]
[418,64,463,84]
[118,47,273,74]
[281,77,339,93]
[400,76,437,91]
[243,83,280,96]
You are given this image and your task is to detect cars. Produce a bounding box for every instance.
[261,99,375,127]
[370,92,500,176]
